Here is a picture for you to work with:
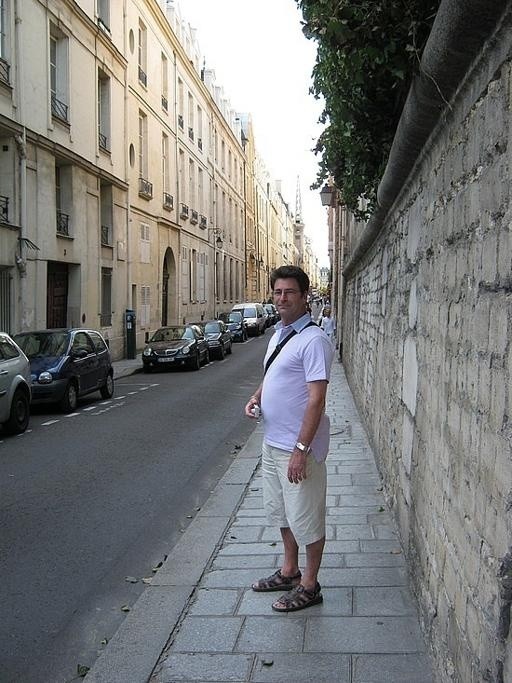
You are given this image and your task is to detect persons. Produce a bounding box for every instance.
[306,298,337,343]
[247,263,338,611]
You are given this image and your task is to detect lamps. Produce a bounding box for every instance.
[319,182,342,207]
[246,248,264,265]
[208,227,223,248]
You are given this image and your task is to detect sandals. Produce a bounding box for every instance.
[251,569,302,593]
[272,581,323,612]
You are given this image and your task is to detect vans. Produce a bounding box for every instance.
[230,302,268,337]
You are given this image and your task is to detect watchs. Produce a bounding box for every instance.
[293,440,313,456]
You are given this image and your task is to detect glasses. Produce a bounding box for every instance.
[270,289,300,297]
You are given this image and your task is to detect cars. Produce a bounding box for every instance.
[11,326,116,413]
[0,333,33,434]
[142,324,210,371]
[262,304,281,327]
[188,319,234,360]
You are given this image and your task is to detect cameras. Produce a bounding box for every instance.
[251,403,260,418]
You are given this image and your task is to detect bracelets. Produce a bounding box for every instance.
[248,393,259,405]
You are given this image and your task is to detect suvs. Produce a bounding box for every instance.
[214,312,249,342]
